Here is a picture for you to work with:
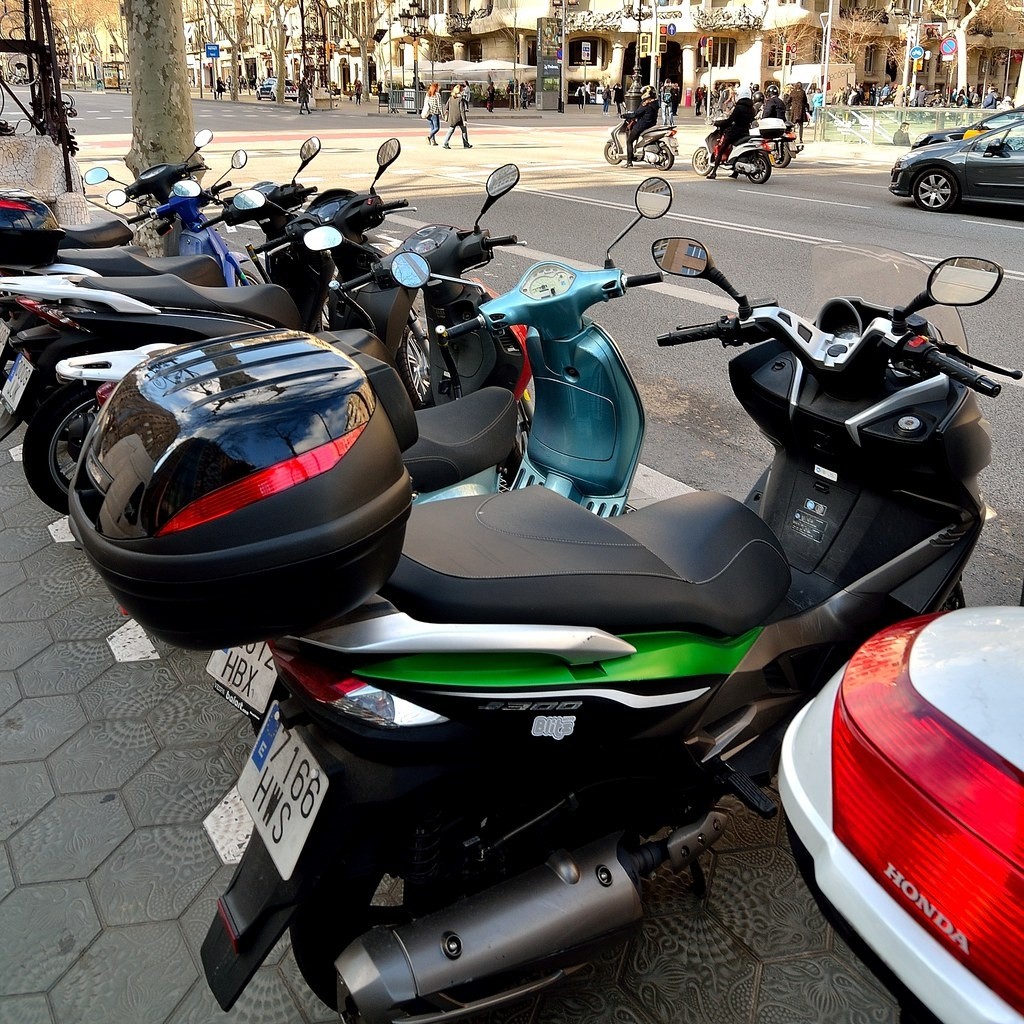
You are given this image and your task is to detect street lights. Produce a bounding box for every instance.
[345,41,352,89]
[623,0,659,113]
[820,10,831,103]
[1002,31,1017,99]
[398,0,430,116]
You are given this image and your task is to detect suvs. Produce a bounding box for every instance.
[256,76,299,102]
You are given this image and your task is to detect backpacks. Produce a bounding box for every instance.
[956,94,964,106]
[971,93,981,108]
[662,86,672,103]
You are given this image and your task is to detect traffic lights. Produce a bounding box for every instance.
[658,22,668,54]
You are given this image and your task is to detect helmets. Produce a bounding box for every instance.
[752,92,765,103]
[640,85,657,101]
[735,86,751,103]
[765,85,780,99]
[753,84,759,92]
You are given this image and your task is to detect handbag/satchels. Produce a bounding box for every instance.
[420,102,431,119]
[444,110,448,122]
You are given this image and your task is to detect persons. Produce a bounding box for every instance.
[216,76,1013,148]
[707,87,755,179]
[421,82,444,146]
[619,86,659,168]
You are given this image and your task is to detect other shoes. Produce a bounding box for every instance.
[432,140,438,146]
[426,136,431,145]
[728,174,737,179]
[621,163,633,168]
[443,143,451,149]
[706,175,715,179]
[464,142,472,148]
[308,111,313,115]
[299,112,304,115]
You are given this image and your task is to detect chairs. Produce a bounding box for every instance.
[378,93,389,113]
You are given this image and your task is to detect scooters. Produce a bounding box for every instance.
[0,119,676,752]
[769,119,805,168]
[64,230,1022,1023]
[691,102,788,185]
[604,102,679,170]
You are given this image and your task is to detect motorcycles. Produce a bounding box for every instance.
[775,603,1024,1024]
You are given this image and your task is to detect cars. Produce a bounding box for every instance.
[888,119,1024,214]
[911,104,1024,151]
